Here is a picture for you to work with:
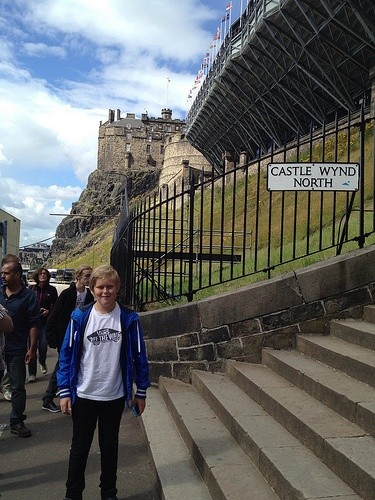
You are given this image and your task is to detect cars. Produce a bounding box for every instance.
[21,265,76,280]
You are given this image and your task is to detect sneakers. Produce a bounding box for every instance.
[42,400,61,413]
[11,421,31,437]
[3,388,12,401]
[28,375,36,383]
[40,363,47,374]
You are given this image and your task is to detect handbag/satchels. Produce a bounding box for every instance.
[49,320,64,348]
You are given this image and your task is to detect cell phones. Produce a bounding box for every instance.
[132,403,140,416]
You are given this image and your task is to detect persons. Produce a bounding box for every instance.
[55,265,151,500]
[0,253,58,433]
[41,266,96,412]
